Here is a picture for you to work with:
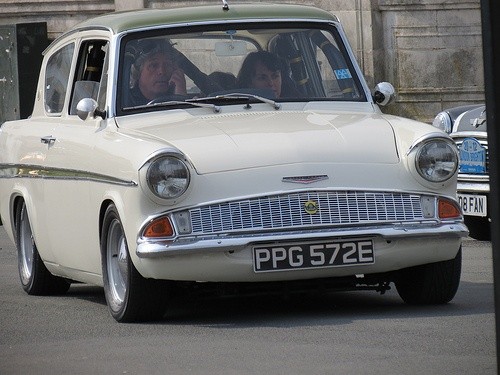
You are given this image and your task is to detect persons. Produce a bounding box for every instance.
[233,50,290,100]
[130,39,187,103]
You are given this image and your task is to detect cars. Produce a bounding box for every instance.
[433,102,491,243]
[0,1,471,325]
